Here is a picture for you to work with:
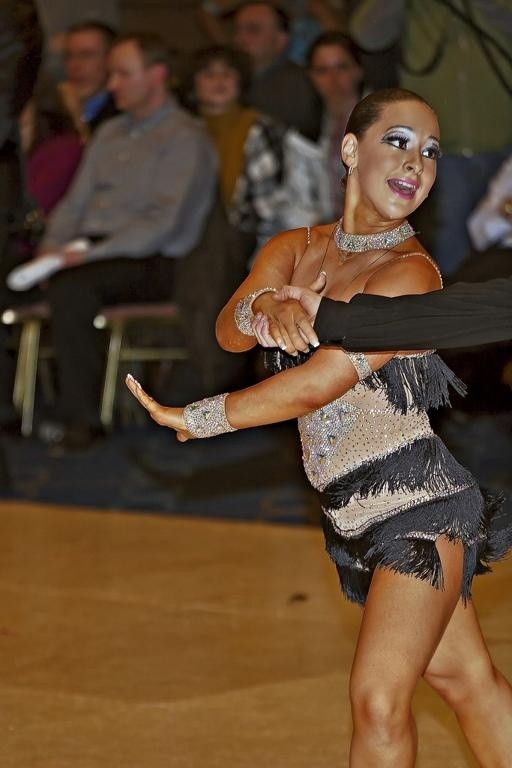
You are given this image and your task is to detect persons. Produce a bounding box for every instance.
[0,0,512,459]
[121,87,511,767]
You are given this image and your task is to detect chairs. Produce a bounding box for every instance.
[0,108,256,440]
